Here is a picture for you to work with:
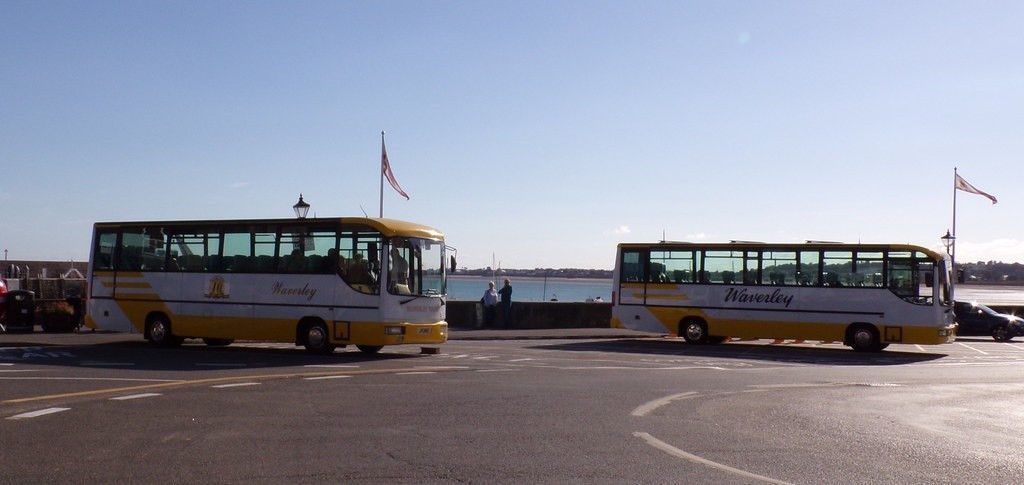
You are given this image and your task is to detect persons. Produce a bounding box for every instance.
[498,278,512,330]
[483,281,498,330]
[388,248,409,295]
[347,254,368,284]
[328,248,336,271]
[290,249,304,274]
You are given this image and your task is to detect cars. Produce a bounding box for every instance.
[954,300,1024,342]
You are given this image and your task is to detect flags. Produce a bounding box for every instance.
[955,172,999,205]
[382,137,411,202]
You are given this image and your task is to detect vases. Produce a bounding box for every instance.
[39,312,78,333]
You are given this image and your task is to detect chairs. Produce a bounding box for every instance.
[258,255,271,271]
[187,255,202,271]
[247,256,257,270]
[202,256,211,271]
[235,255,247,268]
[284,255,295,270]
[272,256,286,271]
[211,255,218,270]
[224,256,235,268]
[309,254,321,273]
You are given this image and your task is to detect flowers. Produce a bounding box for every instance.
[37,302,73,314]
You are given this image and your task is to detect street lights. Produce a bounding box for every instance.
[292,193,311,257]
[940,228,956,254]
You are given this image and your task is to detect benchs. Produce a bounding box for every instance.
[624,262,643,281]
[847,273,865,286]
[722,271,735,284]
[797,272,812,286]
[826,272,843,287]
[747,270,757,284]
[770,271,785,285]
[651,263,670,282]
[673,269,689,282]
[872,273,882,286]
[698,270,711,283]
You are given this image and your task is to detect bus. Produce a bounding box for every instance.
[609,240,955,352]
[84,217,457,355]
